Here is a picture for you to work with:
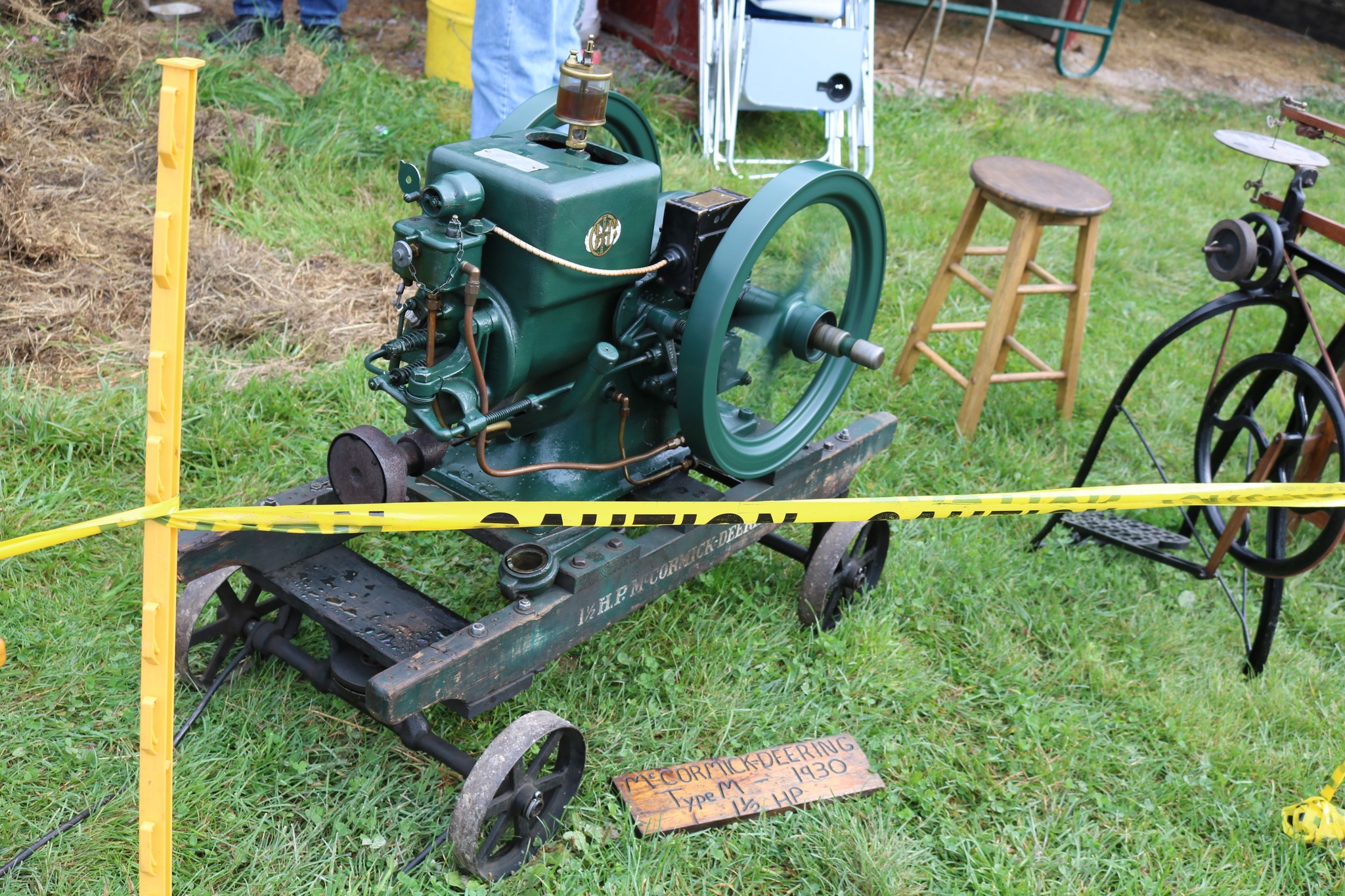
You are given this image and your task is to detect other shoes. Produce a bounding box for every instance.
[306,23,350,60]
[207,12,284,46]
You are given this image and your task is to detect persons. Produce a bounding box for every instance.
[470,0,584,142]
[206,0,352,63]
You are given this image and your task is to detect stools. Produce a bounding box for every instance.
[893,154,1114,446]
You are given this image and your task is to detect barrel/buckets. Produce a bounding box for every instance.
[425,0,476,93]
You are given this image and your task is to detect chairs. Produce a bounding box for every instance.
[698,0,874,179]
